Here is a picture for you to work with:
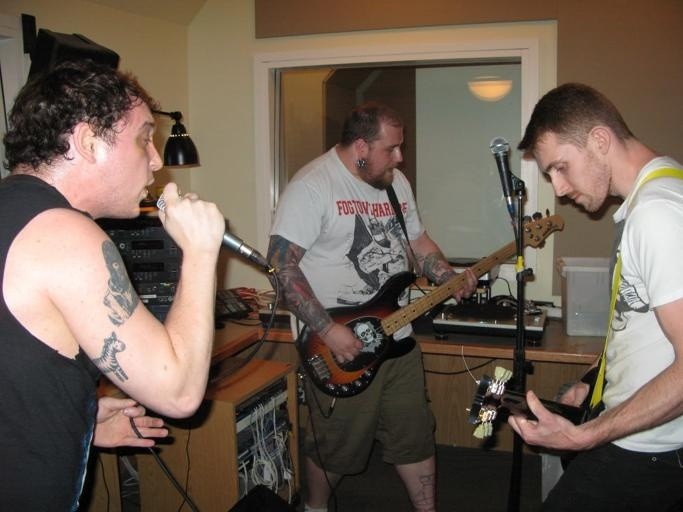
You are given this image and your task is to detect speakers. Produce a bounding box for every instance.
[27,27,121,83]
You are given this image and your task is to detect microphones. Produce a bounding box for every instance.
[156,193,266,267]
[490,136,516,216]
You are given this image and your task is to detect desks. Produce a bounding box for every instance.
[84,313,261,512]
[238,317,606,459]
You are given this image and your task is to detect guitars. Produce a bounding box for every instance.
[468,367,605,469]
[296,210,563,398]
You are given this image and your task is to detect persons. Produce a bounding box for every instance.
[0,59,227,512]
[267,103,479,509]
[505,82,683,512]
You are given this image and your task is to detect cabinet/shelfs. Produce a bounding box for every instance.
[136,359,302,512]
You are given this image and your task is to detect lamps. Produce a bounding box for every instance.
[148,104,200,169]
[466,71,514,104]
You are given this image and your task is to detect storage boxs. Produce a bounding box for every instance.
[554,256,615,338]
